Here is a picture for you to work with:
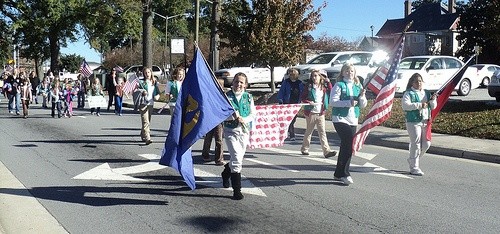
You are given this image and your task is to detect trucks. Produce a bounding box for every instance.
[58,71,84,84]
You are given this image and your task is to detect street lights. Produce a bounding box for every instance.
[154,11,190,73]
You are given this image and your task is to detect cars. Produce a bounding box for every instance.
[472,64,500,88]
[394,55,480,97]
[487,70,500,102]
[213,57,299,89]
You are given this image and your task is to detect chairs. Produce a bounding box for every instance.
[431,63,440,68]
[450,62,456,68]
[419,63,425,68]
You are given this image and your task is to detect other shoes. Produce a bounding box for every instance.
[290,137,296,141]
[217,161,226,165]
[410,168,424,176]
[325,151,337,158]
[202,157,212,162]
[221,172,230,188]
[334,176,354,184]
[146,140,153,145]
[233,192,244,200]
[300,150,310,155]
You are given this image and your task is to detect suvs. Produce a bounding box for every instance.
[323,50,392,90]
[284,52,350,88]
[122,65,163,83]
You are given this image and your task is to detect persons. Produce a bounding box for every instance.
[105,67,117,112]
[0,71,87,118]
[136,66,159,144]
[329,62,367,184]
[301,71,337,158]
[113,72,124,115]
[164,67,185,116]
[202,79,226,165]
[221,72,256,199]
[277,68,304,141]
[91,78,105,116]
[401,73,437,175]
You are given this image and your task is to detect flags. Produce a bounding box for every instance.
[114,65,123,72]
[352,35,405,156]
[79,60,93,78]
[122,74,139,98]
[248,104,302,149]
[159,48,235,193]
[426,59,473,140]
